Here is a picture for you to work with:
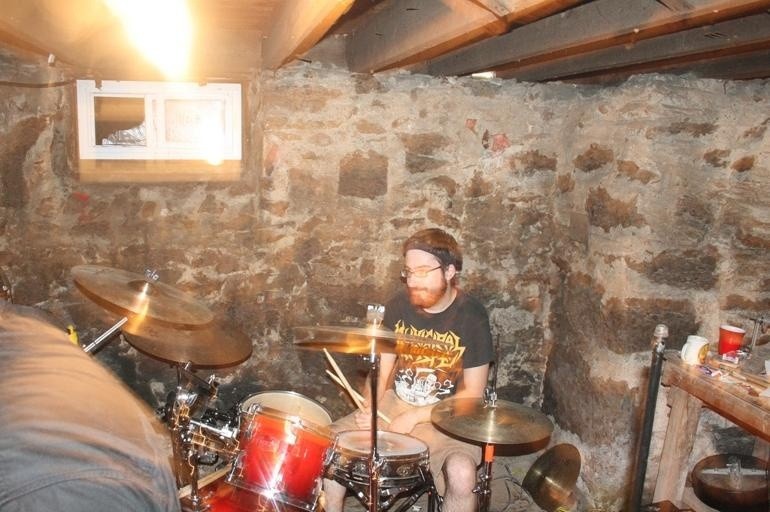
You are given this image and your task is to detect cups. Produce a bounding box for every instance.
[680,334,710,366]
[717,324,745,357]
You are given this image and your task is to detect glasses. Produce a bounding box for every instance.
[400,265,441,278]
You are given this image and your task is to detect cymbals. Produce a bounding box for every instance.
[122,316,252,366]
[431,397,554,445]
[71,264,213,326]
[294,325,453,356]
[521,444,581,511]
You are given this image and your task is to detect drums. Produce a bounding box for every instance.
[326,431,430,487]
[223,403,340,511]
[235,390,334,427]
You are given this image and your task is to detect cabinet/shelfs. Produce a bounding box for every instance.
[651,348,770,512]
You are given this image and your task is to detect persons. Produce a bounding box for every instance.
[0,297,182,511]
[322,227,496,512]
